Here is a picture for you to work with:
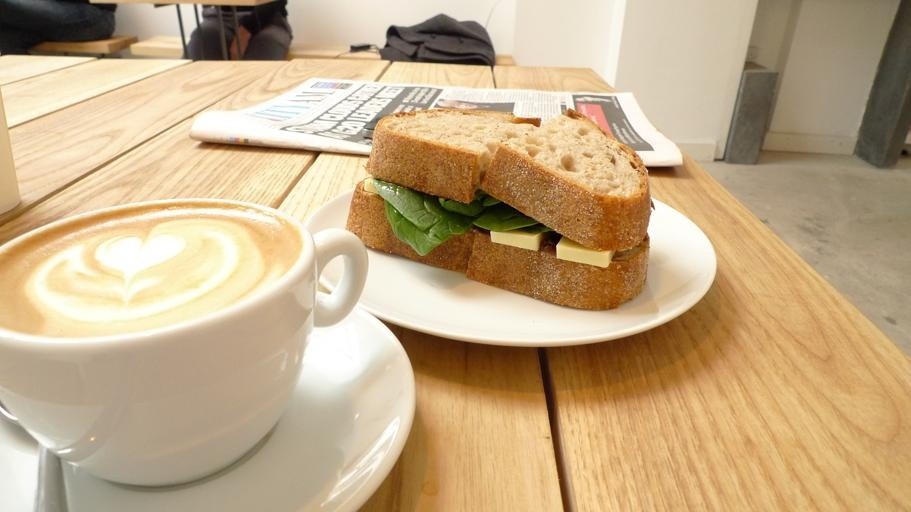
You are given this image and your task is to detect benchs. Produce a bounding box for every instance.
[34,34,517,66]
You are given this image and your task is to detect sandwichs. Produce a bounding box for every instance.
[346,106,650,311]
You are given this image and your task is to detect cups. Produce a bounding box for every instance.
[2,198,367,496]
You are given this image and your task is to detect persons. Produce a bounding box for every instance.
[183,0,294,60]
[0,0,117,54]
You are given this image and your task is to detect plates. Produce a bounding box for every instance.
[2,303,423,512]
[305,180,722,353]
